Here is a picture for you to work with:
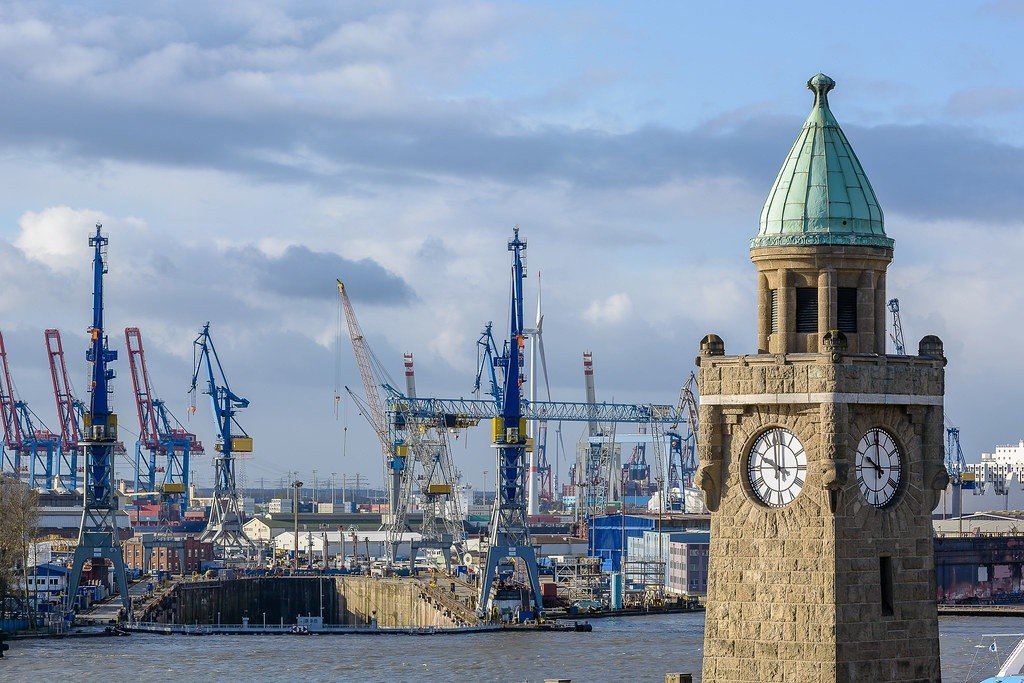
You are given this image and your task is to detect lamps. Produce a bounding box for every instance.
[699,334,724,355]
[822,329,848,351]
[918,335,943,356]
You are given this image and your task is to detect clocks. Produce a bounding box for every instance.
[747,427,808,508]
[854,428,902,508]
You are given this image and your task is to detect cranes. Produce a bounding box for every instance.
[0,221,1024,625]
